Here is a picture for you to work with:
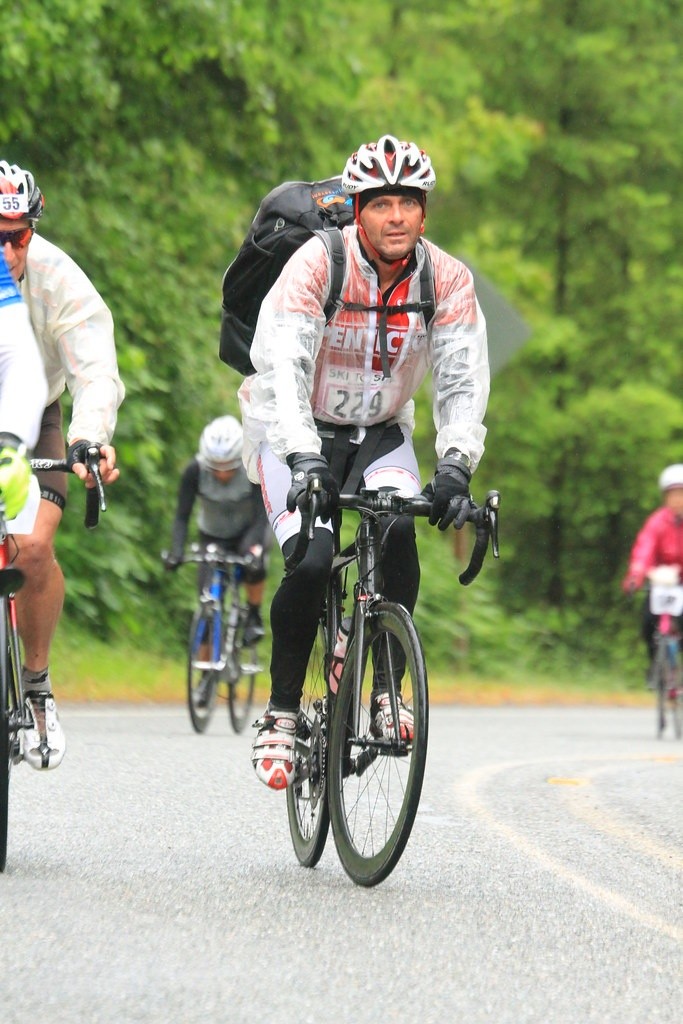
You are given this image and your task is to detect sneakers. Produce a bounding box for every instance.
[252,699,299,790]
[370,692,415,741]
[23,690,66,771]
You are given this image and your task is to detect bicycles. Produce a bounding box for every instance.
[0,445,109,874]
[283,472,500,888]
[629,579,683,739]
[159,543,265,735]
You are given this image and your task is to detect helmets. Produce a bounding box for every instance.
[659,464,683,491]
[340,134,437,197]
[0,160,45,220]
[195,415,243,472]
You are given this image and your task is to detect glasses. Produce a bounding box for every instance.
[0,228,35,251]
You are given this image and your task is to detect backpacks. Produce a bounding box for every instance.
[219,176,437,377]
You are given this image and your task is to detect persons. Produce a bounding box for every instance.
[238,135,490,790]
[621,463,683,690]
[165,417,274,706]
[0,160,126,770]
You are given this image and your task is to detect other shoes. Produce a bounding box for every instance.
[647,669,657,689]
[196,678,213,707]
[243,614,262,645]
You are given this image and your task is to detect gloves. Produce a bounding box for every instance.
[241,543,264,576]
[164,548,183,570]
[616,594,636,612]
[285,453,339,524]
[421,458,478,532]
